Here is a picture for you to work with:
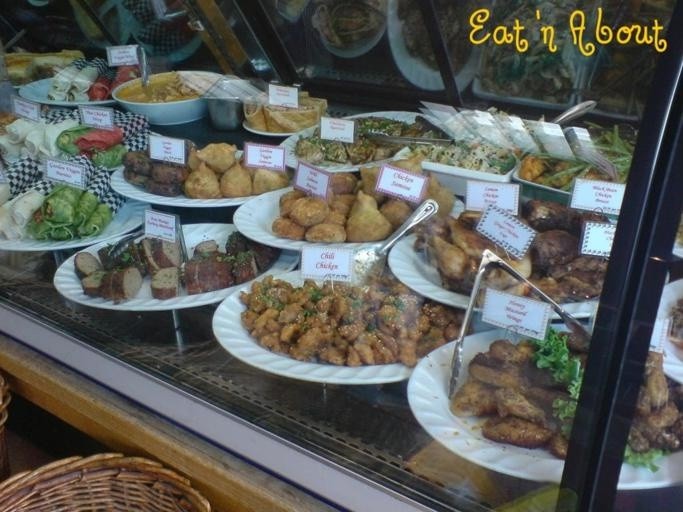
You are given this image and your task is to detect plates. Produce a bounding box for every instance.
[406,324,683,491]
[212,269,500,386]
[107,163,259,210]
[243,111,330,136]
[388,231,671,319]
[53,220,301,313]
[233,183,465,252]
[387,0,490,94]
[313,0,386,59]
[18,75,116,106]
[0,198,153,254]
[278,110,426,172]
[648,278,682,384]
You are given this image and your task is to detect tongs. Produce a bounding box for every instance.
[448,249,591,401]
[362,115,455,149]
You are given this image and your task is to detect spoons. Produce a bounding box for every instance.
[349,199,440,285]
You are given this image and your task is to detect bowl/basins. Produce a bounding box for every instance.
[112,71,224,125]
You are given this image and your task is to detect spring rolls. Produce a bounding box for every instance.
[1,118,126,169]
[45,66,138,102]
[1,186,113,244]
